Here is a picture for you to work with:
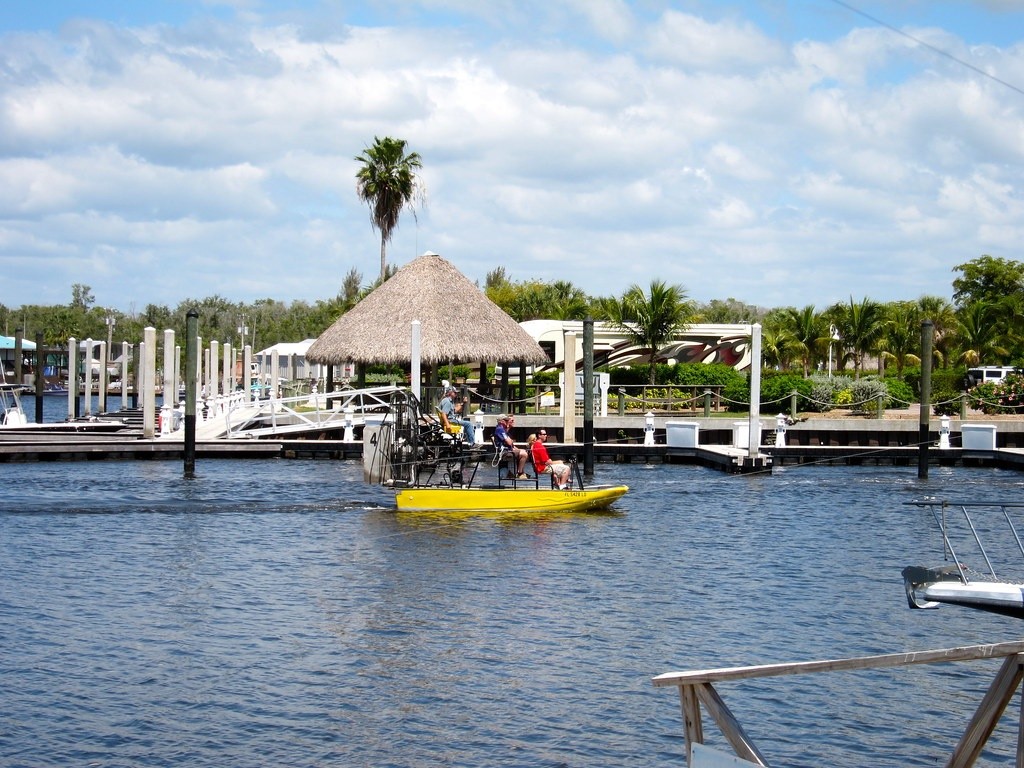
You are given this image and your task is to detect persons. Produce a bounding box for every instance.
[494,413,531,479]
[527,428,570,490]
[439,386,480,448]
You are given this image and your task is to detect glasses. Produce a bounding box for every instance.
[540,434,546,436]
[510,420,514,422]
[505,420,507,421]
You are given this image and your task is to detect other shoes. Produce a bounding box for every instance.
[469,443,483,450]
[559,484,566,490]
[527,474,531,478]
[567,480,573,483]
[518,474,527,479]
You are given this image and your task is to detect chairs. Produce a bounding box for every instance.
[480,388,501,414]
[490,435,539,490]
[526,448,554,490]
[435,406,464,440]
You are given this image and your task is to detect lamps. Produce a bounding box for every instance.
[773,412,788,446]
[938,414,951,448]
[342,403,355,441]
[472,409,485,443]
[643,410,656,444]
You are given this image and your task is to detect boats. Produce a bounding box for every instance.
[0,359,128,432]
[902,502,1024,613]
[387,453,630,516]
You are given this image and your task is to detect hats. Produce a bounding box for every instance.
[446,387,460,392]
[497,414,511,422]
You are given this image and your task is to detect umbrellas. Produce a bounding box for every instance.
[306,251,549,414]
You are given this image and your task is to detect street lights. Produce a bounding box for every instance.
[237,313,250,353]
[103,314,116,414]
[828,324,840,378]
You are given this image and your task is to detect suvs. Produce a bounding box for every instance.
[963,366,1024,393]
